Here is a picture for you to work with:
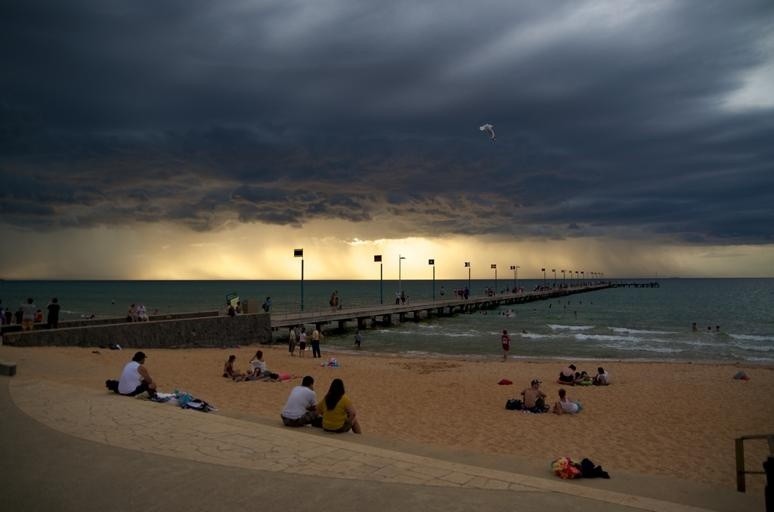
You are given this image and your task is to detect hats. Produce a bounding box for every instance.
[531,379,543,385]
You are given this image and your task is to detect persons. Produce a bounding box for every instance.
[86,314,95,320]
[521,365,611,415]
[330,290,342,312]
[395,290,409,305]
[502,329,511,360]
[481,308,515,318]
[1,297,60,331]
[111,297,115,305]
[280,375,362,433]
[224,351,279,382]
[352,331,362,347]
[692,322,721,333]
[288,325,324,359]
[222,300,241,315]
[118,351,157,397]
[548,299,592,319]
[264,297,272,320]
[127,303,160,322]
[523,329,528,334]
[440,282,568,300]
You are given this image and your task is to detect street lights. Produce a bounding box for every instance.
[492,263,497,292]
[551,268,556,284]
[541,267,545,288]
[580,271,584,284]
[576,271,578,286]
[292,247,304,312]
[399,253,405,295]
[427,258,436,301]
[510,264,516,290]
[587,270,603,285]
[373,253,381,305]
[464,261,471,290]
[561,269,565,285]
[569,270,572,286]
